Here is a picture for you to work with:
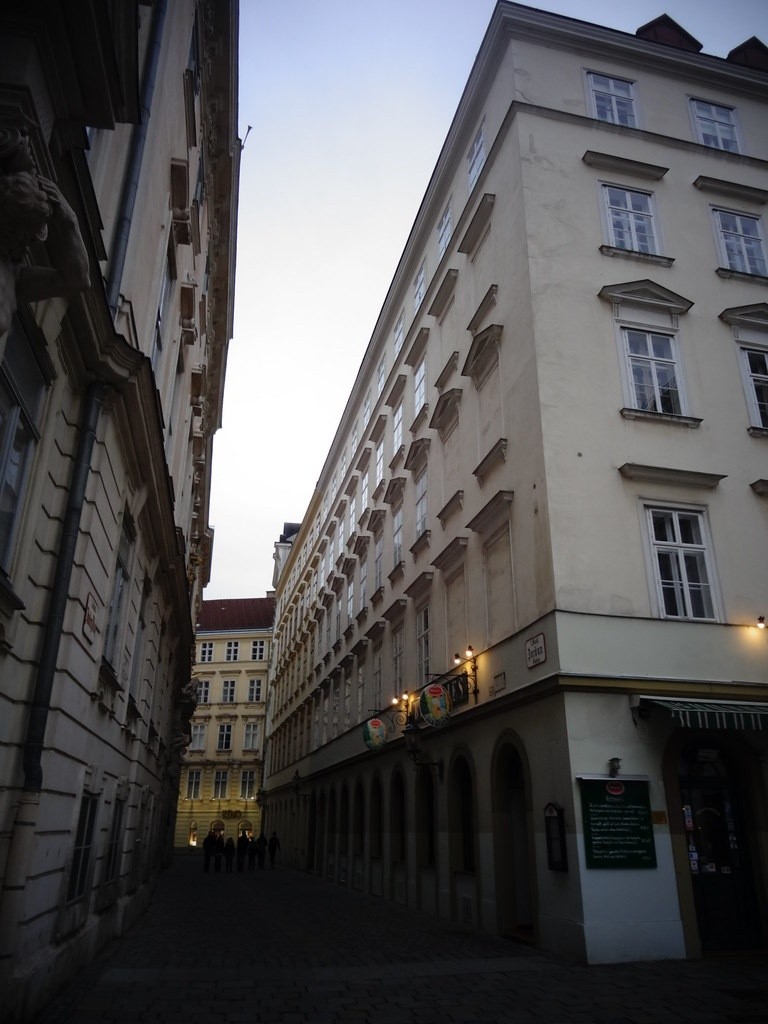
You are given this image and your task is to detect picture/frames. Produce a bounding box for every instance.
[544,802,568,873]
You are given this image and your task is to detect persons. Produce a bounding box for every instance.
[215,834,225,871]
[202,831,216,874]
[236,829,248,873]
[247,837,258,871]
[223,837,235,873]
[267,830,282,868]
[256,833,268,872]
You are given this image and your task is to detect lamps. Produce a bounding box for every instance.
[392,690,409,726]
[454,645,480,705]
